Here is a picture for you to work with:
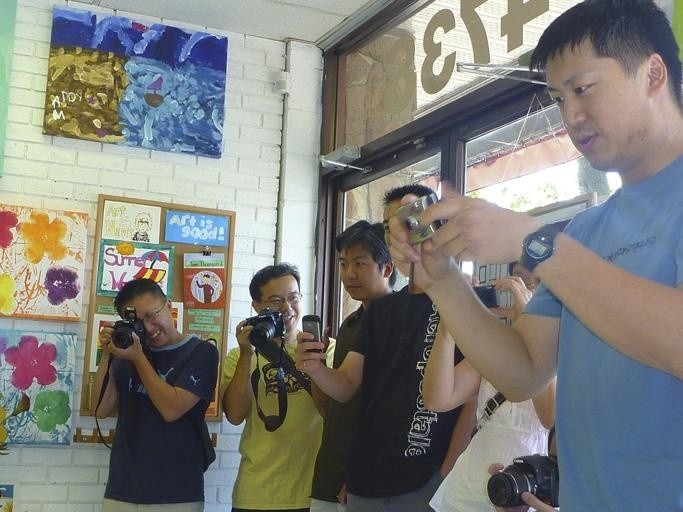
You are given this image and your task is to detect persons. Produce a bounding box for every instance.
[488,424,559,512]
[195,274,214,304]
[295,186,479,512]
[422,262,556,512]
[218,265,336,512]
[132,212,153,242]
[91,278,219,512]
[308,220,398,512]
[387,0,683,512]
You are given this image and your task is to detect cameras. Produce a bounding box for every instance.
[473,285,499,308]
[395,194,445,246]
[111,305,146,349]
[487,454,560,508]
[245,308,285,347]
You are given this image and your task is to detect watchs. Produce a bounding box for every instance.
[519,224,561,272]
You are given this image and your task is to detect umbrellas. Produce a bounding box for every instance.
[142,251,168,269]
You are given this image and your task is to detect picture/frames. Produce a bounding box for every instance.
[81,194,236,424]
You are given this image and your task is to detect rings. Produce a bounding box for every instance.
[302,360,306,367]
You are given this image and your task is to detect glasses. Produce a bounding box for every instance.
[258,293,302,309]
[141,298,168,325]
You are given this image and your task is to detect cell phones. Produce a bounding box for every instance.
[303,316,323,353]
